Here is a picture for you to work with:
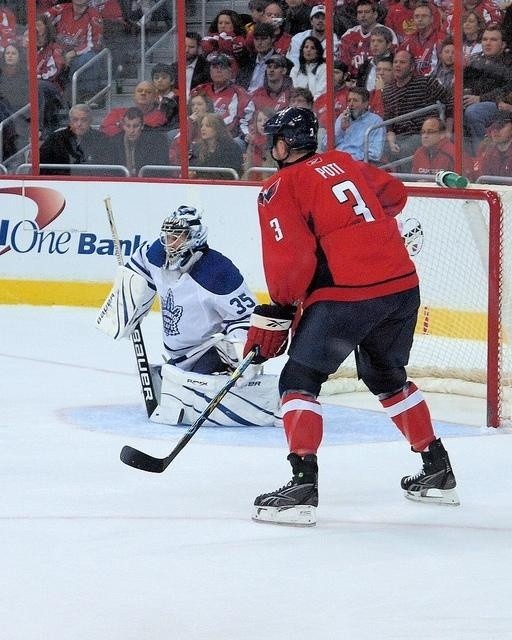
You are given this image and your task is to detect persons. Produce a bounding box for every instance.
[243,108,457,511]
[97,206,281,428]
[0,0,511,184]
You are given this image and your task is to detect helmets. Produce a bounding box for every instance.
[160,206,207,256]
[264,106,318,148]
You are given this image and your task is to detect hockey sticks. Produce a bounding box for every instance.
[103,194,185,425]
[120,352,254,473]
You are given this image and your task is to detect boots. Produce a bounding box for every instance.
[401,438,456,491]
[254,453,318,506]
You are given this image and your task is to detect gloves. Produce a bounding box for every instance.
[244,304,297,365]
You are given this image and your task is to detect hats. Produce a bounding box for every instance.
[207,51,231,63]
[254,24,274,37]
[265,55,286,68]
[310,5,325,18]
